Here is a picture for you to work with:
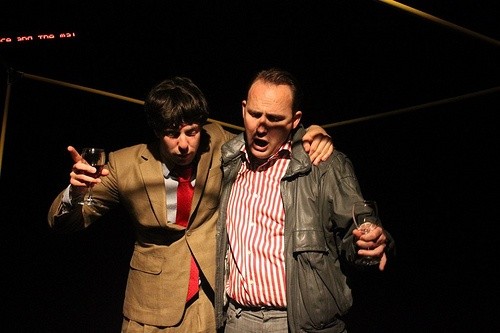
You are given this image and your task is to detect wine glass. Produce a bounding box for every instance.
[352,201,378,265]
[78,148,106,206]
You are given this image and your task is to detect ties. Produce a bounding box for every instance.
[174,167,201,303]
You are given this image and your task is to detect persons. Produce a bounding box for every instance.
[215,71,394,333]
[48,77,333,333]
